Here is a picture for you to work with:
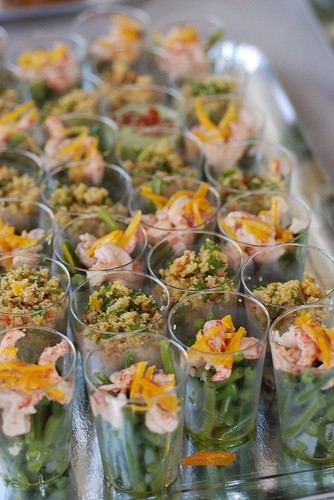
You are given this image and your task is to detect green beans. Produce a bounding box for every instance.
[0,24,334,500]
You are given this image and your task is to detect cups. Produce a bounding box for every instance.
[1,4,334,494]
